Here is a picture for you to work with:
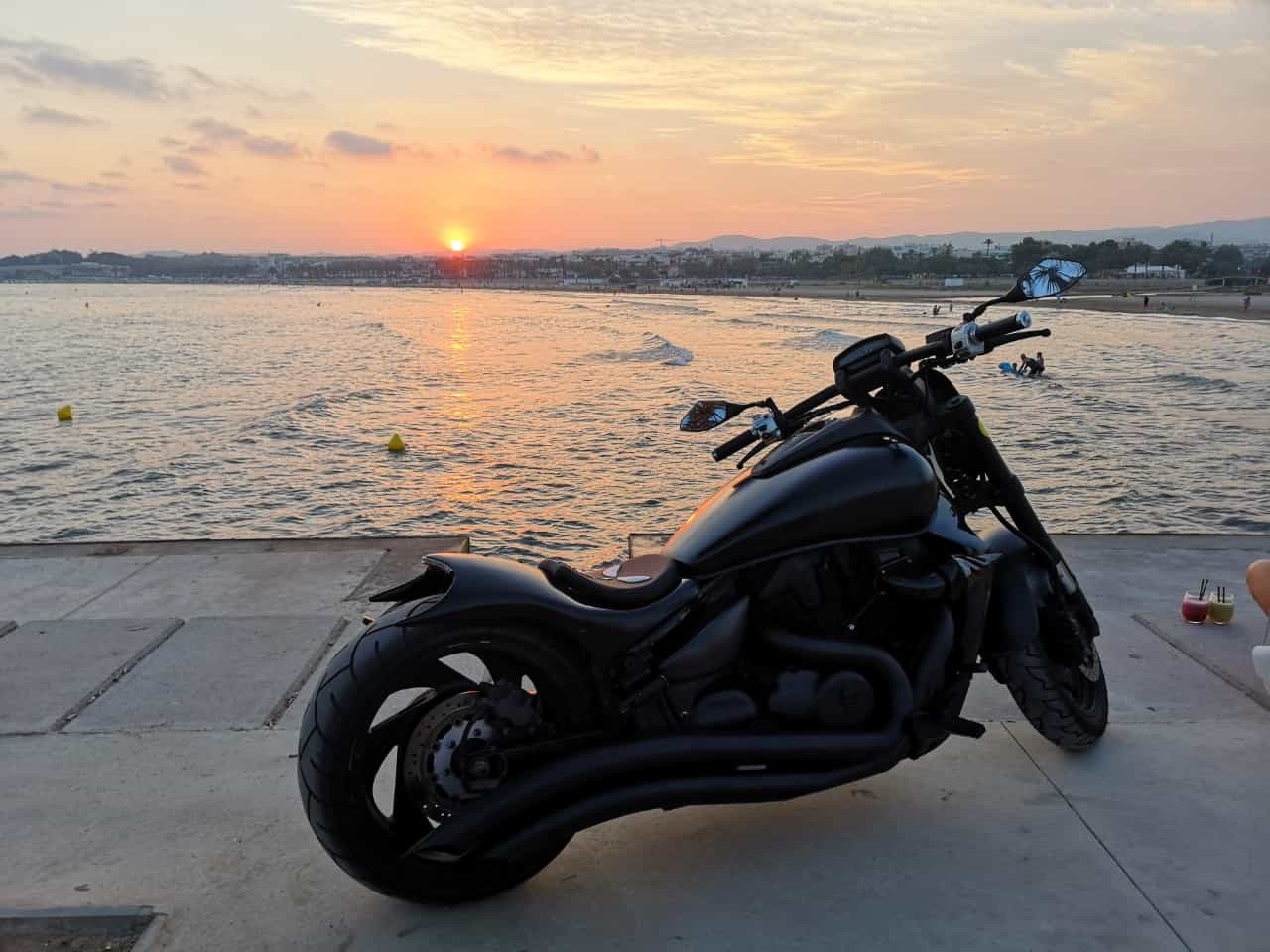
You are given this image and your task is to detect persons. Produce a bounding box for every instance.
[1013,351,1045,378]
[1246,558,1270,696]
[1143,295,1149,308]
[933,303,953,315]
[1243,293,1250,311]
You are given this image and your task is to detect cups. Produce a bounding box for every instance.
[1207,591,1236,626]
[1180,589,1208,624]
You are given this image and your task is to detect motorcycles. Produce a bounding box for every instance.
[295,257,1113,906]
